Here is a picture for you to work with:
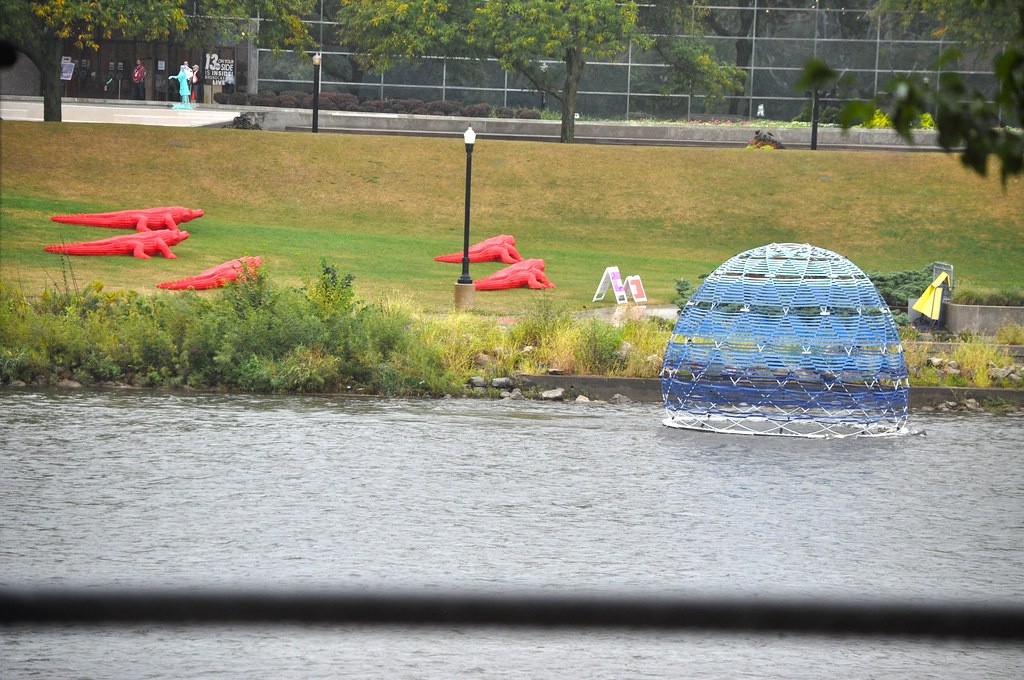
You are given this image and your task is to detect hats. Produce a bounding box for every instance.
[192,65,199,70]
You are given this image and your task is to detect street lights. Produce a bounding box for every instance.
[458,125,475,284]
[311,53,321,133]
[922,78,929,116]
[541,63,549,112]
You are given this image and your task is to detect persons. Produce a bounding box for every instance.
[168,61,203,110]
[130,58,146,100]
